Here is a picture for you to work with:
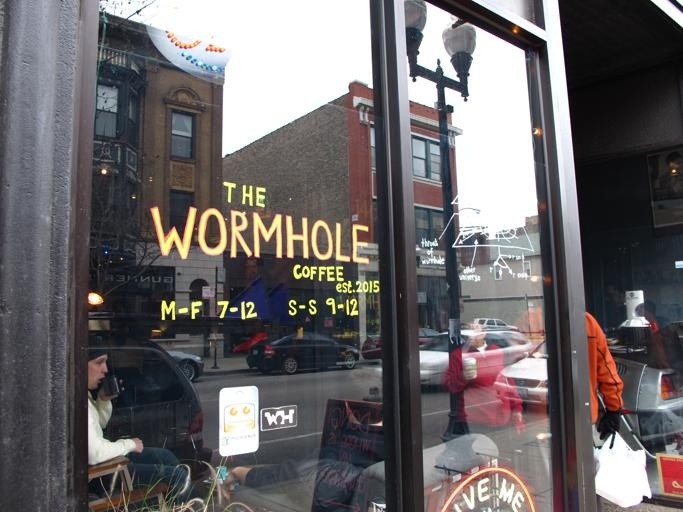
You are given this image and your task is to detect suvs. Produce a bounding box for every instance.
[472,316,520,331]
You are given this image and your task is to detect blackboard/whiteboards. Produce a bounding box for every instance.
[311,398,386,512]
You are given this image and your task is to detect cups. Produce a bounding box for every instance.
[216,467,226,480]
[462,358,477,380]
[102,374,121,397]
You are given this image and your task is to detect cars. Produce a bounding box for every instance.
[88,328,212,483]
[418,329,550,417]
[245,331,361,375]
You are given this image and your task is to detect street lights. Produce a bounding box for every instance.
[400,0,478,441]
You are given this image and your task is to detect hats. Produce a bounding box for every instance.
[88,333,107,361]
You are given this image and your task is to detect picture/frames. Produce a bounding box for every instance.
[644,147,682,239]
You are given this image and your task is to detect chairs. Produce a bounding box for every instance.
[228,432,498,511]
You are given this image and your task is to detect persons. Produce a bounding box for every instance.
[221,367,386,511]
[440,312,524,474]
[87,332,201,512]
[505,272,625,512]
[635,302,682,389]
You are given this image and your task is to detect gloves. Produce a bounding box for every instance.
[512,416,527,436]
[598,407,621,448]
